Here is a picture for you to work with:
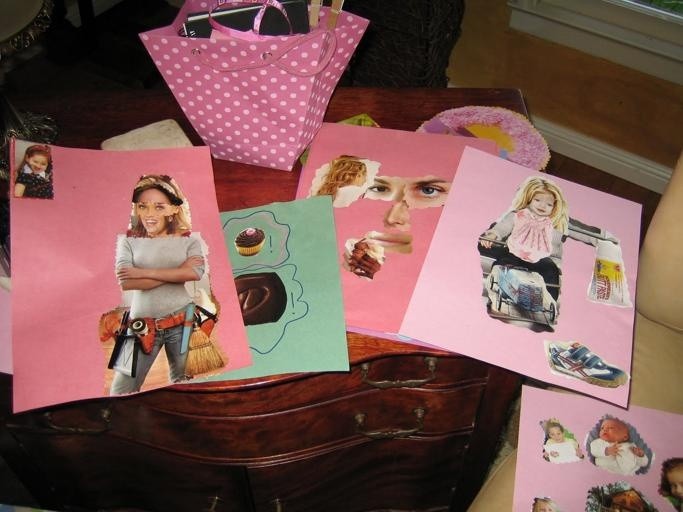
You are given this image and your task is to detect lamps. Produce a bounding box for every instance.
[0,1,60,177]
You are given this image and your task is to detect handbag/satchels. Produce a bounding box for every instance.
[138,0,371,172]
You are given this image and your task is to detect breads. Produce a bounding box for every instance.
[234,271,288,326]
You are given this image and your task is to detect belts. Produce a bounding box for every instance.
[127,311,187,329]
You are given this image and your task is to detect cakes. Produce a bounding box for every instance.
[234,228,265,256]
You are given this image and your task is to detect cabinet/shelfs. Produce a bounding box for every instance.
[0,84,548,511]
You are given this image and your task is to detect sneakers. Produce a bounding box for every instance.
[543,337,627,389]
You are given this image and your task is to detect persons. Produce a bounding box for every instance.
[532,415,683,512]
[480,176,618,321]
[318,154,454,254]
[110,176,208,395]
[12,144,55,200]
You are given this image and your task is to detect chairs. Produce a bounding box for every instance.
[466,145,683,510]
